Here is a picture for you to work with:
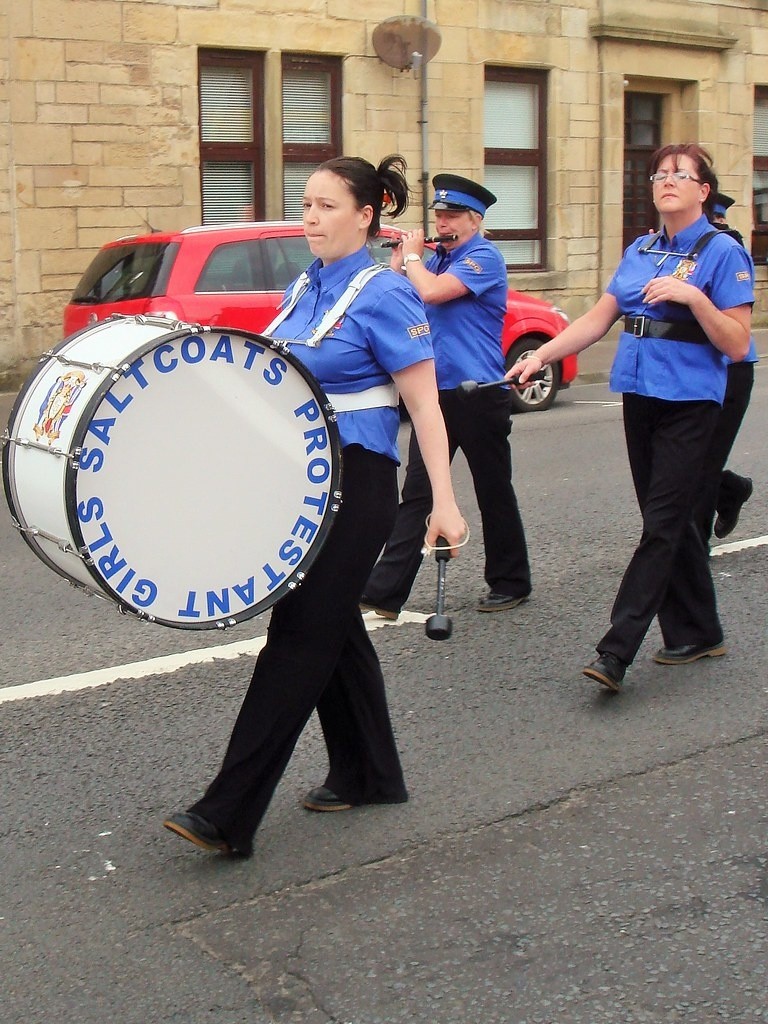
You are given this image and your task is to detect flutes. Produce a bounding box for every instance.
[380,234,459,249]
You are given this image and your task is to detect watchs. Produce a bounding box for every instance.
[400,253,422,270]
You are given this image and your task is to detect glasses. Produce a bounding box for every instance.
[650,173,704,187]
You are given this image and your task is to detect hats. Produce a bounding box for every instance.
[422,171,497,221]
[708,192,735,219]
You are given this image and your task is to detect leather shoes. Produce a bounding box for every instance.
[653,636,725,665]
[357,593,401,621]
[713,474,753,540]
[476,591,529,612]
[304,783,354,811]
[584,651,626,694]
[163,807,234,860]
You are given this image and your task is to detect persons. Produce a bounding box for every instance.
[164,159,407,861]
[504,144,756,694]
[356,174,532,622]
[648,191,755,539]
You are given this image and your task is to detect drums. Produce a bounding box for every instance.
[1,311,344,634]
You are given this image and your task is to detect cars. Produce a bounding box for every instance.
[62,192,580,418]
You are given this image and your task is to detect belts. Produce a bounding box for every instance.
[620,315,710,347]
[321,383,402,412]
